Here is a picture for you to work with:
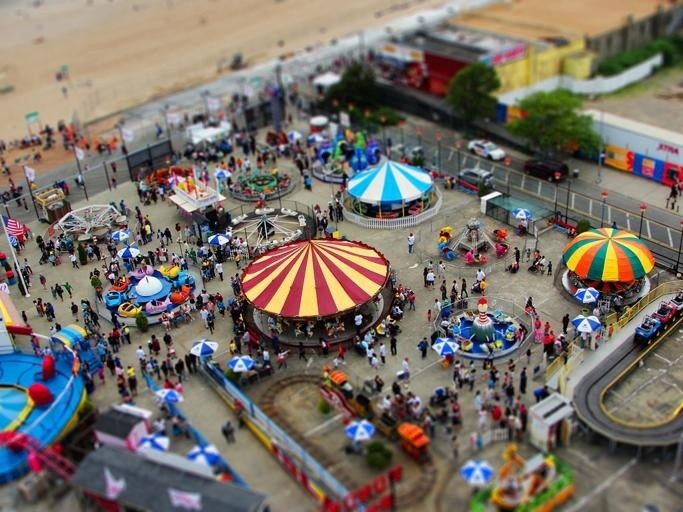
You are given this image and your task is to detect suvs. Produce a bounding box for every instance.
[457,167,497,190]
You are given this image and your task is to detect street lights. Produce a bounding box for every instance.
[584,89,606,184]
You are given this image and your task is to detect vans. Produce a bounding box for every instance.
[523,154,568,184]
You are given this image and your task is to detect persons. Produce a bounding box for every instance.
[0,49,682,512]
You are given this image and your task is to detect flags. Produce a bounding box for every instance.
[24,167,36,181]
[165,112,179,124]
[207,95,220,109]
[75,147,84,160]
[2,217,26,241]
[168,490,201,510]
[121,128,132,142]
[102,468,127,499]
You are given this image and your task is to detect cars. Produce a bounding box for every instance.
[466,138,507,162]
